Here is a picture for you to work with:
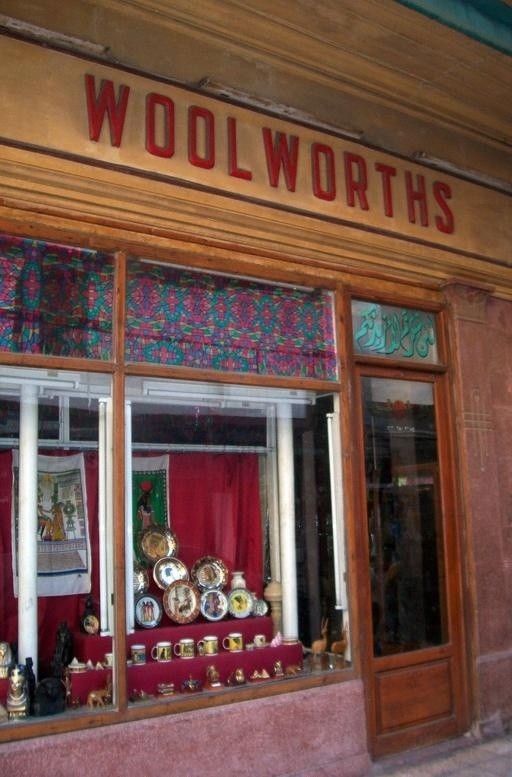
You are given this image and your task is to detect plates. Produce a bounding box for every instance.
[133,526,268,629]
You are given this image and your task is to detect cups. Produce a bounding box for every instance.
[105,632,264,665]
